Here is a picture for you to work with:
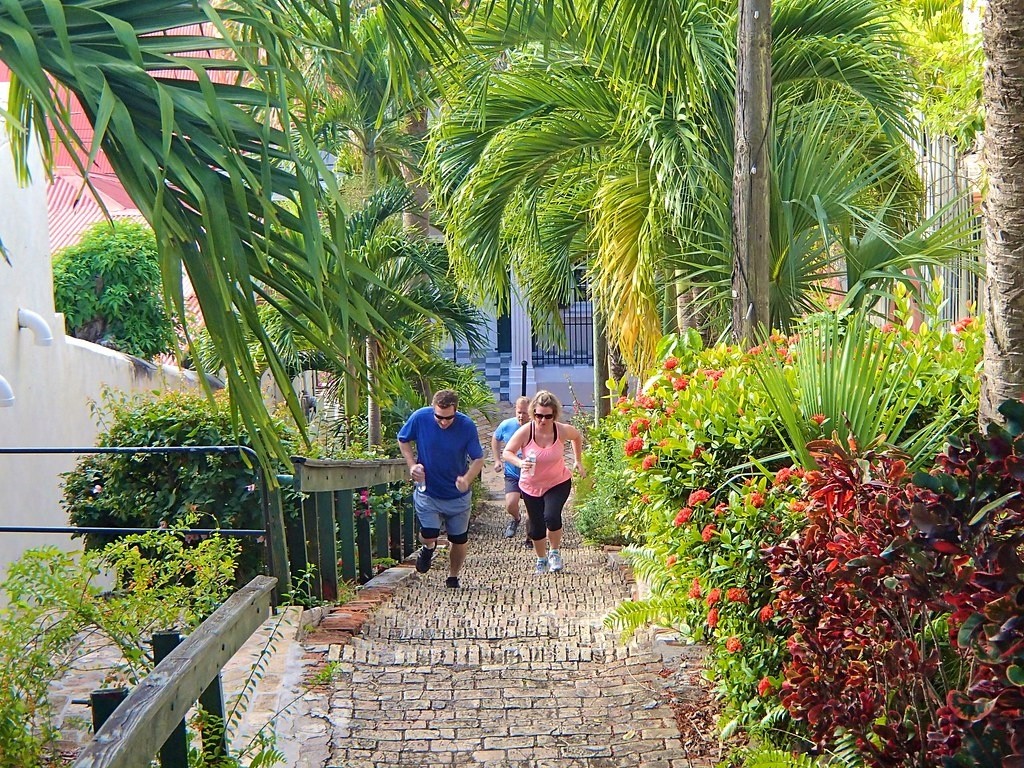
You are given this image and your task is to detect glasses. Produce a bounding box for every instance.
[434,413,455,420]
[534,413,555,419]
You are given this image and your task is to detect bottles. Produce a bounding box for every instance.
[528,449,536,476]
[417,481,426,492]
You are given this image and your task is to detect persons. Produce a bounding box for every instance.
[491,396,534,550]
[397,389,485,588]
[502,390,586,571]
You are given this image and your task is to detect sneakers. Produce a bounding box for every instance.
[415,540,437,573]
[524,538,534,550]
[506,514,521,537]
[445,576,460,589]
[535,557,549,574]
[548,550,563,571]
